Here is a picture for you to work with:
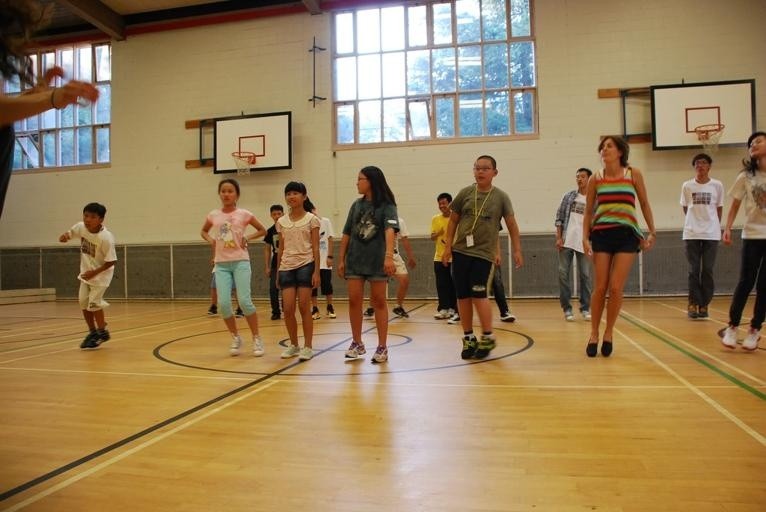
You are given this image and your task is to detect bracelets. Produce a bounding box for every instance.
[386,250,395,260]
[327,255,334,260]
[49,89,61,113]
[650,232,656,241]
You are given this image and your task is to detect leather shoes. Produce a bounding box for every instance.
[602,335,613,357]
[587,337,599,357]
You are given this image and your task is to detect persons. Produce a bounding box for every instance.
[553,167,593,324]
[441,155,524,360]
[581,135,657,359]
[304,200,339,320]
[57,202,116,347]
[676,153,724,320]
[200,178,268,356]
[274,180,321,360]
[364,217,416,318]
[429,192,460,320]
[446,222,515,325]
[337,165,400,364]
[262,204,287,323]
[721,130,766,352]
[0,3,100,221]
[208,234,245,320]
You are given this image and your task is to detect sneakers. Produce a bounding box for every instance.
[392,306,409,319]
[687,304,709,321]
[234,308,244,318]
[281,345,300,359]
[363,308,375,320]
[742,327,762,350]
[500,312,516,322]
[207,305,218,315]
[252,338,265,356]
[345,341,366,359]
[310,305,337,320]
[298,347,314,360]
[475,336,497,358]
[722,325,738,349]
[460,335,477,359]
[370,345,389,364]
[434,308,461,324]
[230,336,242,356]
[564,310,592,322]
[271,313,281,320]
[80,329,110,349]
